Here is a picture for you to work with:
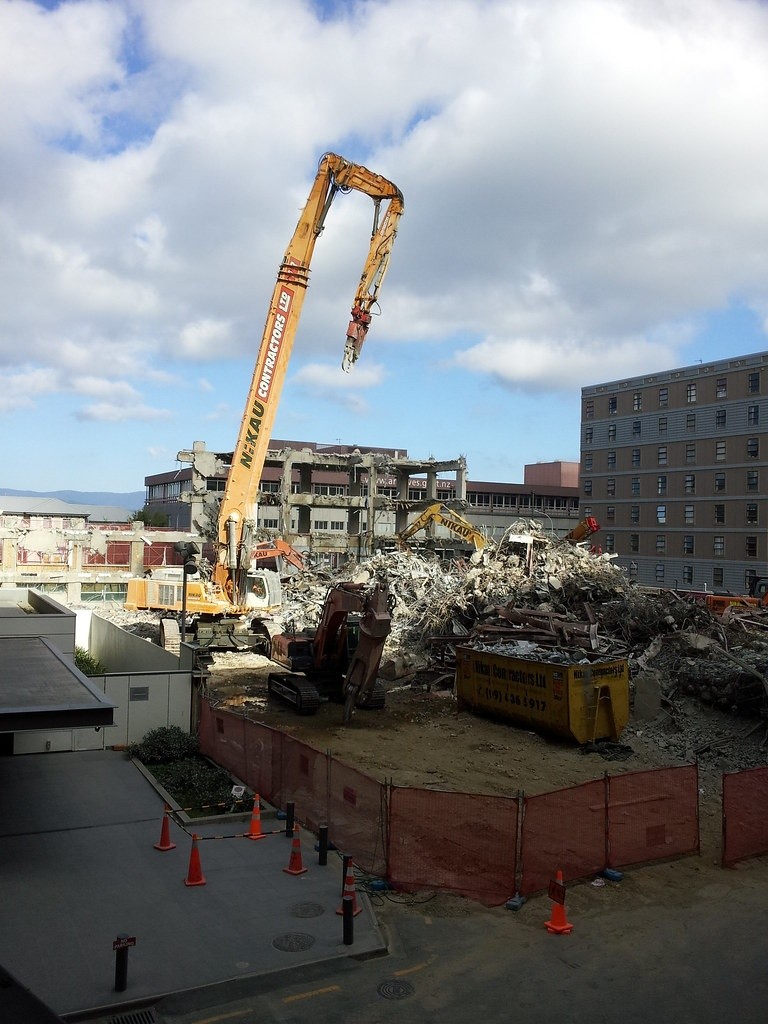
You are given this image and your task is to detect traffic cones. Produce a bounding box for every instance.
[154,805,177,850]
[336,860,364,917]
[244,794,266,839]
[183,834,208,887]
[283,823,308,874]
[541,869,573,935]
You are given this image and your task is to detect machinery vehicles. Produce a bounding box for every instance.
[382,502,601,568]
[268,584,397,728]
[122,149,406,664]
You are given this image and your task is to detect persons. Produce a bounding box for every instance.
[252,581,265,596]
